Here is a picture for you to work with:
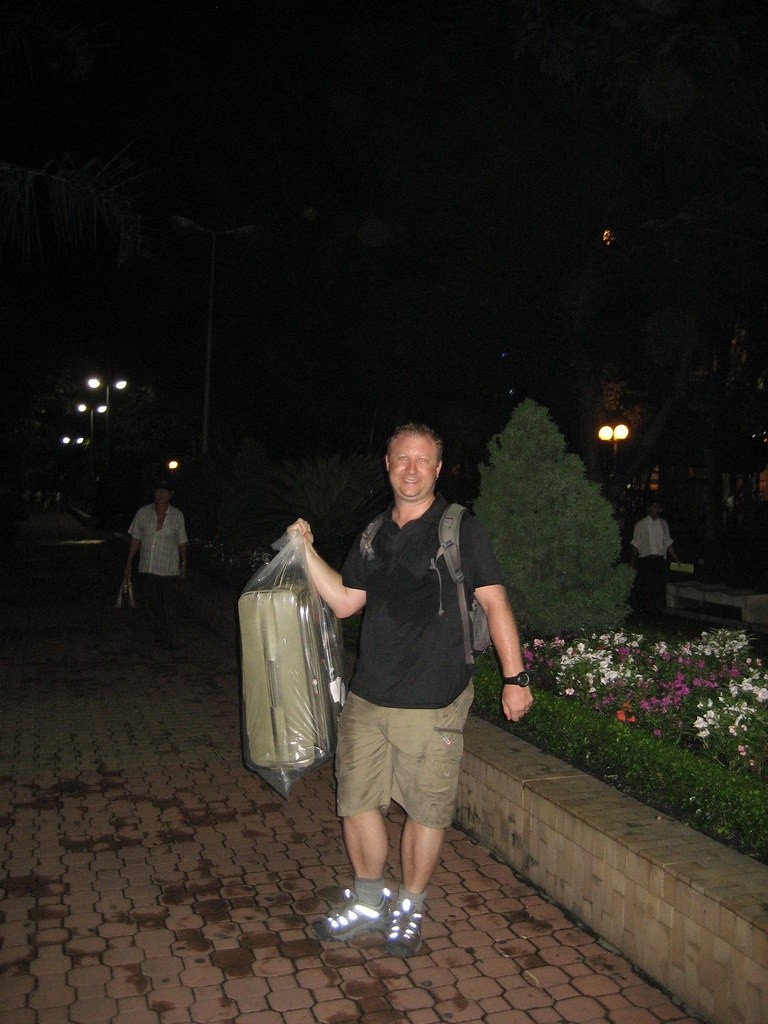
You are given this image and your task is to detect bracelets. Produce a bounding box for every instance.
[181,561,186,565]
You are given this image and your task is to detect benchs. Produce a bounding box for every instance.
[666,581,768,625]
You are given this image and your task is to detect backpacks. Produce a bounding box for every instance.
[357,502,492,666]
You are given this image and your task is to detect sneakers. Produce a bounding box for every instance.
[312,891,394,942]
[385,900,424,956]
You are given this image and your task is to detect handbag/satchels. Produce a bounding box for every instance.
[114,576,135,609]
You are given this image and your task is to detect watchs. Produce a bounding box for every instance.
[503,671,530,688]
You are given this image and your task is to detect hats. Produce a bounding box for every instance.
[152,477,173,490]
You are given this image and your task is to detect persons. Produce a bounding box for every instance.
[124,476,188,652]
[629,497,682,629]
[286,423,535,959]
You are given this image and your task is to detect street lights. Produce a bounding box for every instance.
[598,424,629,478]
[87,376,128,466]
[78,403,107,452]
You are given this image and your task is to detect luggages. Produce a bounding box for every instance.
[237,579,344,769]
